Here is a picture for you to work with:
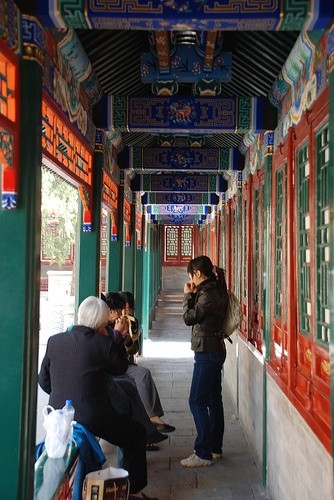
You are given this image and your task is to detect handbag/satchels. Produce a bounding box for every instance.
[43,404,72,459]
[82,466,129,500]
[223,289,243,338]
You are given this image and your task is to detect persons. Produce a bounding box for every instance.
[180,256,228,468]
[38,291,176,500]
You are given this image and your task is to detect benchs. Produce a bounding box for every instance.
[34,436,102,500]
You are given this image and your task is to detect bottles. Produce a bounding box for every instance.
[62,399,75,444]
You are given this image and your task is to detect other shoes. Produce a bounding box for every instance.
[151,421,176,433]
[180,453,212,467]
[148,432,168,444]
[129,492,157,500]
[212,452,222,459]
